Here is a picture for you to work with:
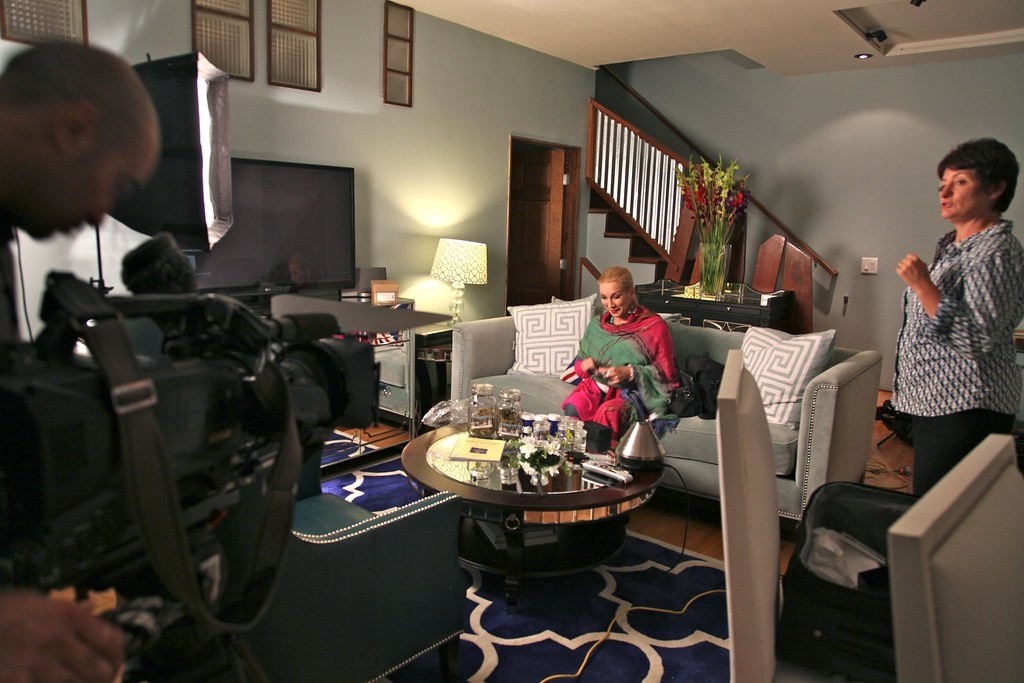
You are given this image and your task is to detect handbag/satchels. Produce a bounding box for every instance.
[669,351,725,419]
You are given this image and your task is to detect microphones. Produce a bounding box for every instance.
[266,313,337,344]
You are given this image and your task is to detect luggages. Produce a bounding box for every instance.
[774,481,924,683]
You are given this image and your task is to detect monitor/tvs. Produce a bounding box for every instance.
[194,157,355,297]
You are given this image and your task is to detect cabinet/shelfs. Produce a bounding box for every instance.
[287,295,454,425]
[633,279,798,333]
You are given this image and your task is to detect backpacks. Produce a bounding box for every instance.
[0,273,331,633]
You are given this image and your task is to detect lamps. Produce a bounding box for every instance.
[432,237,488,326]
[866,29,888,44]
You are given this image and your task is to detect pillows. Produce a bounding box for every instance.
[728,324,838,428]
[502,296,596,378]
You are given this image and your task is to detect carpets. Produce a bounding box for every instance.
[316,429,777,683]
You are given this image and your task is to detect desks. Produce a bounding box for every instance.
[416,325,454,435]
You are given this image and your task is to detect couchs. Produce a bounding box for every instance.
[203,443,473,683]
[453,301,884,524]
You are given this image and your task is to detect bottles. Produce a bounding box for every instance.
[521,412,588,452]
[468,384,522,438]
[466,459,494,480]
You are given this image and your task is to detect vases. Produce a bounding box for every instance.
[697,244,728,295]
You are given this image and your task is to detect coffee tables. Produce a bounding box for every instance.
[401,415,667,614]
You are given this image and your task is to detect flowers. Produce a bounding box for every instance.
[516,434,566,477]
[674,154,751,248]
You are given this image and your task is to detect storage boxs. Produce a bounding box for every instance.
[370,280,399,306]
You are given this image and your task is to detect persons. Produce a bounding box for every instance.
[563,267,680,440]
[288,253,314,282]
[891,138,1024,498]
[0,40,162,683]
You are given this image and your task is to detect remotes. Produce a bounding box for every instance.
[581,461,633,485]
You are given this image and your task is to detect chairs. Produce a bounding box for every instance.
[715,352,868,683]
